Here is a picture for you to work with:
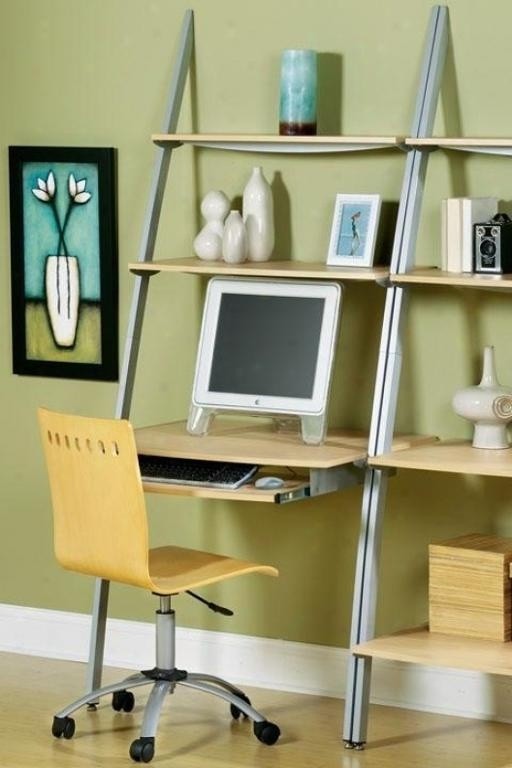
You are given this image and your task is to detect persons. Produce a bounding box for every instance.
[349,244,361,256]
[352,216,361,245]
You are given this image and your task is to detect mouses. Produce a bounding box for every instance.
[253,474,287,491]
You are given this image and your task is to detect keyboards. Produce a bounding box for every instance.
[139,455,262,489]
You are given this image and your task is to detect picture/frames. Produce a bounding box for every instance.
[8,145,119,383]
[326,193,382,266]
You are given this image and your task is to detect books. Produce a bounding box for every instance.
[440,194,501,275]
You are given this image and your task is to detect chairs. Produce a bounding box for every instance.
[33,405,281,763]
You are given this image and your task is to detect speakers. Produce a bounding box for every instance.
[474,213,512,274]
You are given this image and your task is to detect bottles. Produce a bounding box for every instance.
[192,166,275,263]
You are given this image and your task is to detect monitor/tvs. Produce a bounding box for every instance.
[187,278,346,444]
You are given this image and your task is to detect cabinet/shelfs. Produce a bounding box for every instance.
[351,8,512,754]
[83,8,441,750]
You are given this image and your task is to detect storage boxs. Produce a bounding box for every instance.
[428,532,512,645]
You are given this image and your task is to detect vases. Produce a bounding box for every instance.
[222,210,247,263]
[242,166,275,262]
[194,189,232,262]
